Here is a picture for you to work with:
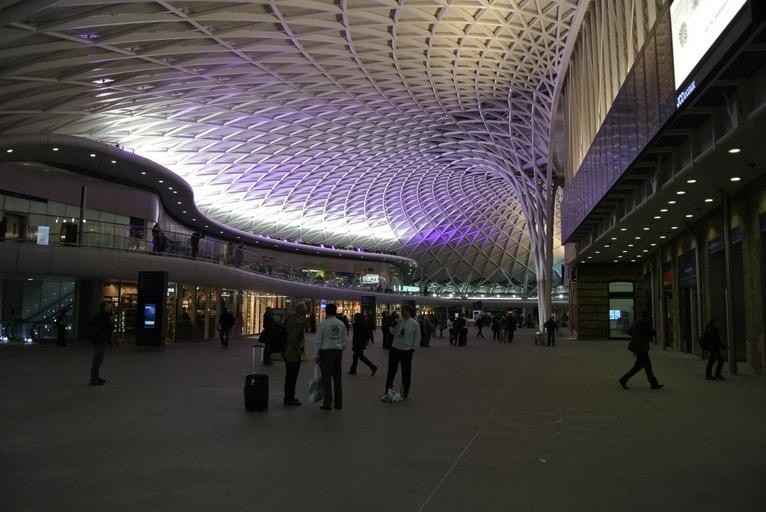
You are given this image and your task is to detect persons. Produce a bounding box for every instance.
[151,222,163,254]
[423,283,429,295]
[89,300,114,385]
[190,232,206,260]
[217,306,235,349]
[262,300,533,410]
[235,244,247,268]
[544,316,559,346]
[619,308,664,390]
[226,240,233,265]
[53,309,68,347]
[704,316,728,380]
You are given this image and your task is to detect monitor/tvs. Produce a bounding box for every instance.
[609,309,620,320]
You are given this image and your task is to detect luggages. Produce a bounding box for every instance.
[244,345,269,411]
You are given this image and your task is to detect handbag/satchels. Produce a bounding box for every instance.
[308,377,325,403]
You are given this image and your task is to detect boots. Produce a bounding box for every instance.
[91,367,106,385]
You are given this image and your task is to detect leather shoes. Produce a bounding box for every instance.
[284,398,301,406]
